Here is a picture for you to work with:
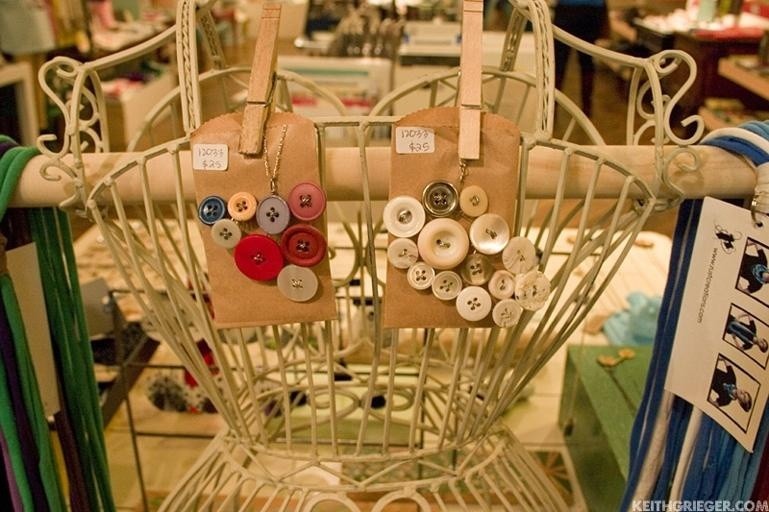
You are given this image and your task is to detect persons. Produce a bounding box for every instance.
[553,0,611,120]
[725,312,768,353]
[710,356,752,412]
[739,242,769,295]
